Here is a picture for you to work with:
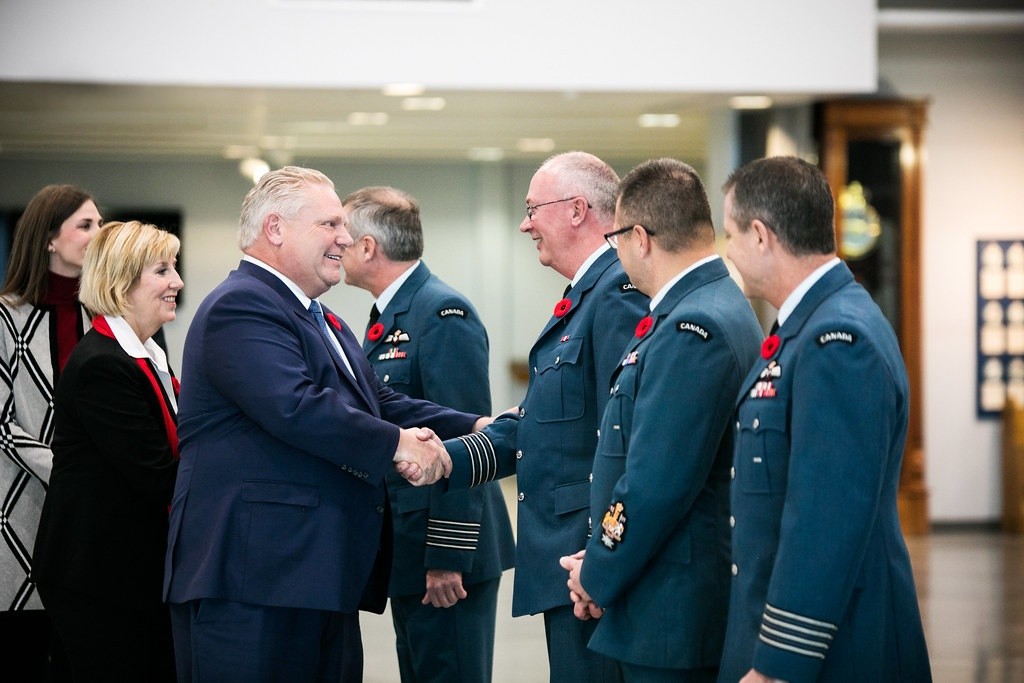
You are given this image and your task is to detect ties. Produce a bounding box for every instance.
[768,319,781,338]
[561,283,573,299]
[368,302,380,333]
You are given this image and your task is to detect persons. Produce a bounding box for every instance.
[170,163,522,683]
[720,152,931,683]
[33,221,186,683]
[2,184,102,683]
[345,184,517,683]
[559,158,763,683]
[396,147,648,683]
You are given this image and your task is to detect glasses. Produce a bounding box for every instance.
[604,223,656,252]
[525,196,594,218]
